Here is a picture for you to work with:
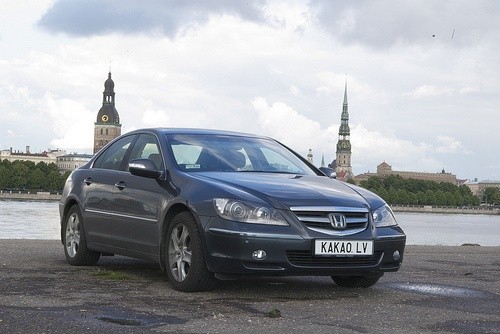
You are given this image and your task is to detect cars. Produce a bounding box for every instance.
[57,127,405,291]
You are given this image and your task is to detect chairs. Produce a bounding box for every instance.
[113,149,246,175]
